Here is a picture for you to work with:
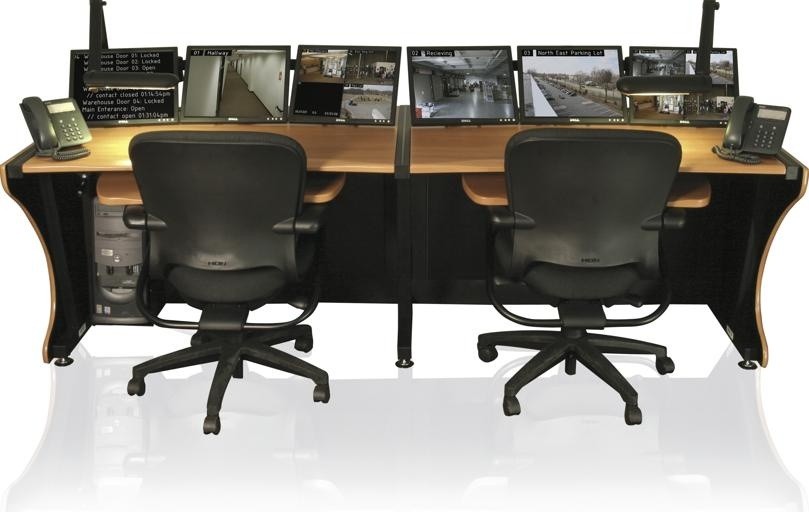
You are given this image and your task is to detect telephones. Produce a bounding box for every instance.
[723,96,791,154]
[19,97,92,154]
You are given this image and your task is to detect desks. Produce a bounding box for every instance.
[0,104,809,372]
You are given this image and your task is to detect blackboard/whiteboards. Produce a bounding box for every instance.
[69,46,179,128]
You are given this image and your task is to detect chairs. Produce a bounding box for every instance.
[474,128,685,427]
[121,124,331,439]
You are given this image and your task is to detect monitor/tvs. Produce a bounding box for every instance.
[69,47,179,127]
[407,46,519,126]
[629,46,739,127]
[179,45,291,123]
[517,45,628,125]
[289,45,401,126]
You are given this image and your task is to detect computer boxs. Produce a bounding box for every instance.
[88,197,168,326]
